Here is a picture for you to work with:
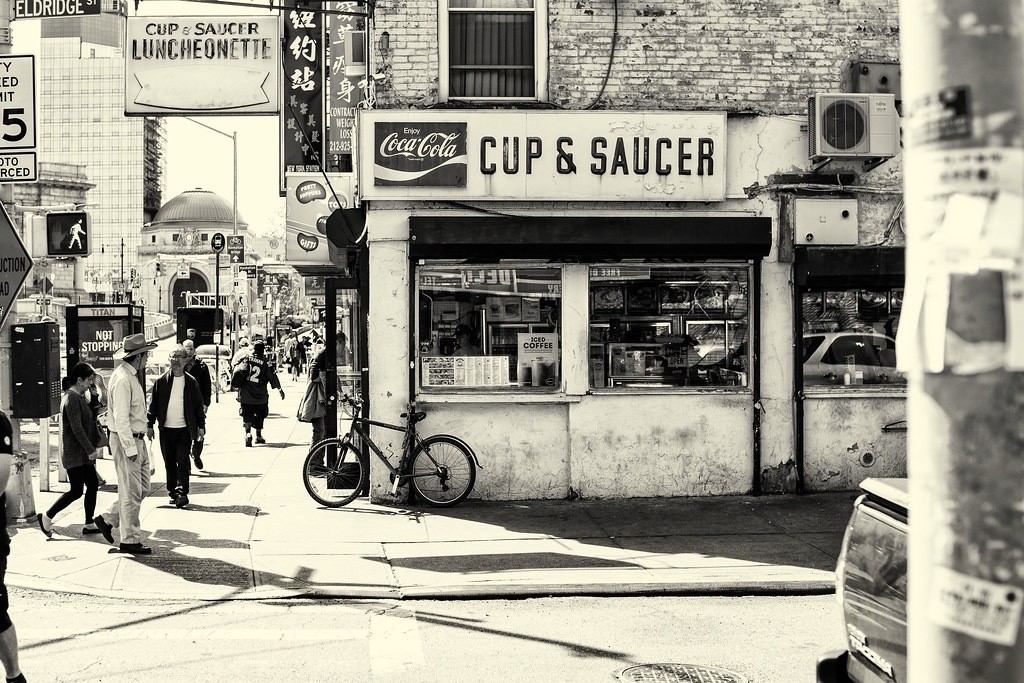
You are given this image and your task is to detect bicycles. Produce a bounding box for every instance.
[301,393,484,508]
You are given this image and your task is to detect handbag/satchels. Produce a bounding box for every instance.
[231,354,250,388]
[93,420,109,447]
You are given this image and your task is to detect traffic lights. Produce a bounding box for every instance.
[161,262,168,276]
[45,211,92,257]
[154,262,162,277]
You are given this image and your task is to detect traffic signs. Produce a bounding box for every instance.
[225,235,245,265]
[177,263,190,279]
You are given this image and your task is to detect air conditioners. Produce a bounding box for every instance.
[807,94,901,158]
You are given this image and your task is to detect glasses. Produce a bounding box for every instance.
[170,355,188,360]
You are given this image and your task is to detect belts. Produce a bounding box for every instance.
[133,433,144,439]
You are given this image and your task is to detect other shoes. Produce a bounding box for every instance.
[174,493,189,507]
[308,465,327,477]
[83,527,101,534]
[37,513,52,537]
[246,436,251,446]
[190,453,203,469]
[255,436,265,443]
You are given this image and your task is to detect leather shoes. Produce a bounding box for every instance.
[94,515,114,543]
[120,543,151,553]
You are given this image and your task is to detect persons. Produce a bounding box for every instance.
[182,340,212,475]
[308,333,346,478]
[147,345,207,506]
[231,338,285,447]
[289,336,312,382]
[451,324,484,356]
[0,406,28,683]
[93,333,158,554]
[36,361,101,537]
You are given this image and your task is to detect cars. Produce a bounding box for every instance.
[803,332,907,386]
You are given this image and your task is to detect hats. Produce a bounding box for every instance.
[239,338,248,346]
[451,324,471,337]
[113,333,158,360]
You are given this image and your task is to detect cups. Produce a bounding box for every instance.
[854,370,863,385]
[522,367,532,387]
[531,360,546,386]
[844,371,851,385]
